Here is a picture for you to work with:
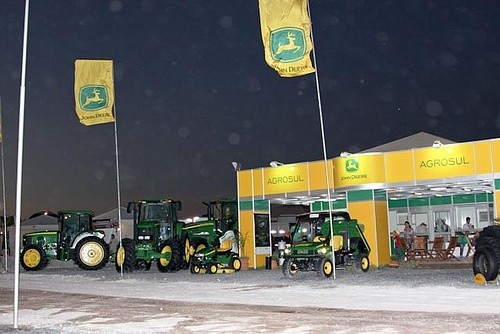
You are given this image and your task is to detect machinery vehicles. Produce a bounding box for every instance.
[282,211,371,279]
[115,197,190,273]
[19,208,110,270]
[181,199,238,254]
[190,239,242,274]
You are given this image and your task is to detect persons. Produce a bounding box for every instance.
[108,235,119,262]
[1,234,9,263]
[402,221,415,248]
[463,217,474,231]
[417,219,450,243]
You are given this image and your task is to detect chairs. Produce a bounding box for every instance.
[399,237,460,262]
[313,235,343,251]
[217,240,232,252]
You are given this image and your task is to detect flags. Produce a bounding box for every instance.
[74,59,114,127]
[257,0,316,79]
